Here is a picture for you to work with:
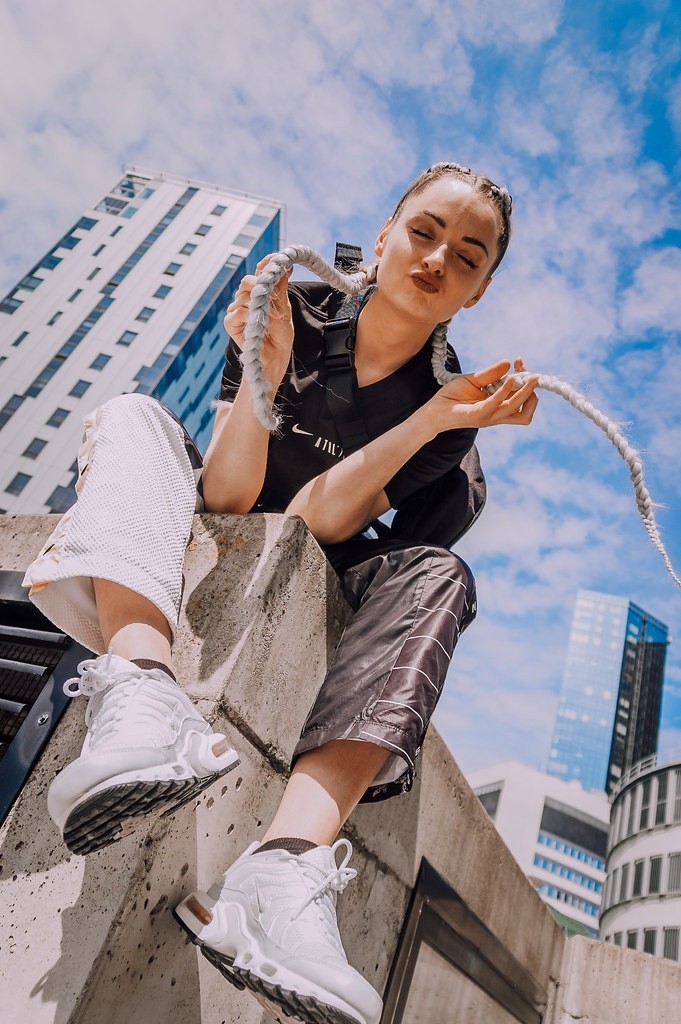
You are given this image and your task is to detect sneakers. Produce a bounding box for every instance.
[46,646,242,856]
[171,839,383,1024]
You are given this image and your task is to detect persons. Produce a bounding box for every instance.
[20,161,541,1024]
